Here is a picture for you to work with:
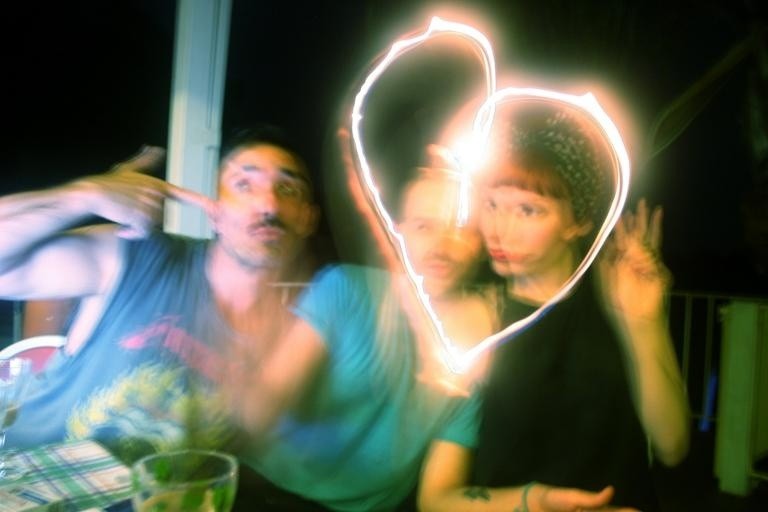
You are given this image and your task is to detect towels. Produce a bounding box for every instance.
[0,438,138,512]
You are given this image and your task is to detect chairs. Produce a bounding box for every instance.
[0,334,66,389]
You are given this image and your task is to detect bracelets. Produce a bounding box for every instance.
[522,479,543,511]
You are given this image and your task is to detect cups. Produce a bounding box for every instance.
[131,451,239,512]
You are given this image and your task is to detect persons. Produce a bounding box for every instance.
[1,138,316,512]
[209,164,639,512]
[461,135,698,512]
